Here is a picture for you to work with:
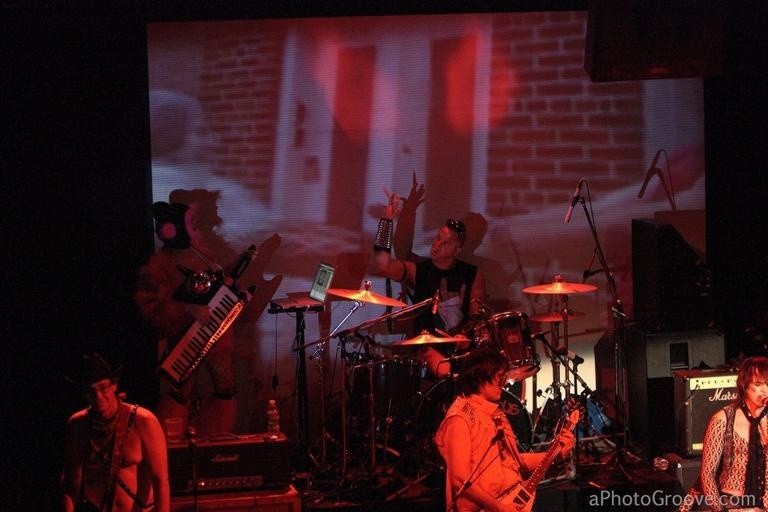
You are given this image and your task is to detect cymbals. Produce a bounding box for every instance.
[324,286,409,309]
[524,280,598,296]
[532,309,588,322]
[391,334,473,347]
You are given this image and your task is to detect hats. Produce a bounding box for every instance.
[68,352,123,386]
[151,202,197,252]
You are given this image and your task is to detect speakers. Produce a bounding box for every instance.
[626,321,728,450]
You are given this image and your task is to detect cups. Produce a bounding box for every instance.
[165,418,183,445]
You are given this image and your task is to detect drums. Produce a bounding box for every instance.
[356,356,421,404]
[414,374,533,488]
[374,410,412,463]
[475,310,540,381]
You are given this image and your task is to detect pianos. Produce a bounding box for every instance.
[158,245,258,386]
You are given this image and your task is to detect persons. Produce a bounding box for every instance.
[59,352,172,511]
[679,357,768,512]
[432,349,578,512]
[131,201,237,443]
[360,217,486,379]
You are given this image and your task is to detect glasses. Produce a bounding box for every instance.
[86,385,110,397]
[495,376,506,386]
[446,219,464,239]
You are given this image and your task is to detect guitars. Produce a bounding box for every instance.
[478,400,586,512]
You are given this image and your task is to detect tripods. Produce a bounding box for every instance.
[312,373,365,506]
[531,364,603,489]
[601,307,644,482]
[304,358,341,493]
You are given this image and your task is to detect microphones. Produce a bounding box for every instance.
[637,145,662,198]
[557,345,587,363]
[500,387,523,407]
[760,394,768,405]
[563,179,584,221]
[360,280,375,312]
[181,426,199,450]
[429,287,443,316]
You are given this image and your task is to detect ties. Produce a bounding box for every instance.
[739,400,767,508]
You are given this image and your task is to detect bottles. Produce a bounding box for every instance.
[267,400,280,440]
